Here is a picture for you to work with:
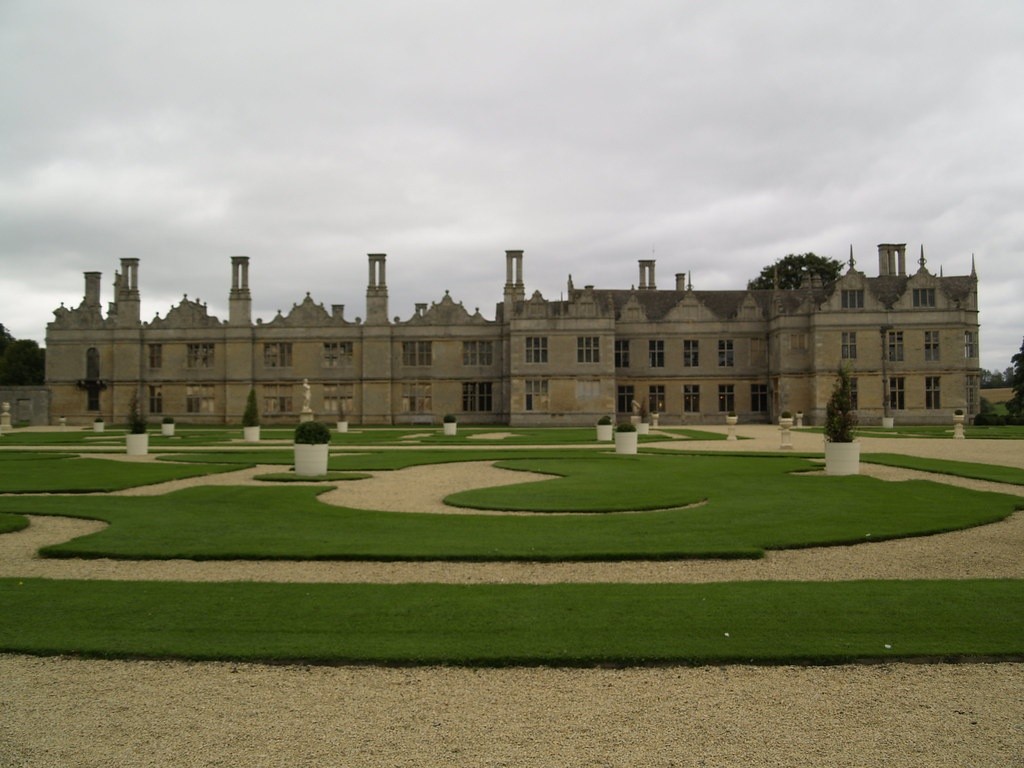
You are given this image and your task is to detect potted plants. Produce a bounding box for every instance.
[94,418,105,432]
[161,417,175,436]
[294,420,332,476]
[59,416,67,426]
[882,394,894,428]
[778,410,794,449]
[443,414,457,436]
[615,423,638,454]
[241,388,260,440]
[796,410,803,427]
[126,388,150,455]
[725,411,738,440]
[336,402,349,432]
[636,397,649,434]
[596,416,613,441]
[823,359,861,477]
[651,411,659,427]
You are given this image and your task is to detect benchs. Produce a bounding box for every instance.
[411,416,433,425]
